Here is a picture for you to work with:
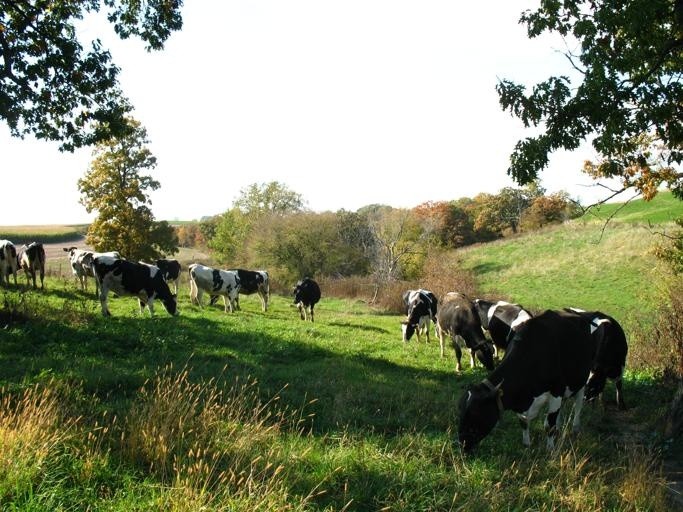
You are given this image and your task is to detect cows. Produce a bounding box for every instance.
[187,263,243,314]
[399,287,630,456]
[0,238,46,290]
[61,246,181,319]
[291,274,323,321]
[209,266,271,312]
